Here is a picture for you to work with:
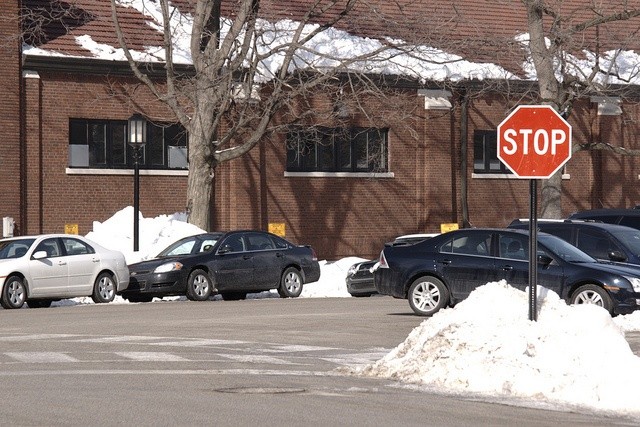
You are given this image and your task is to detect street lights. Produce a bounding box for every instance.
[126,112,148,251]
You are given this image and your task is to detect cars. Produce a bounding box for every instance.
[374,227,640,316]
[345,233,486,298]
[0,234,130,309]
[116,230,320,302]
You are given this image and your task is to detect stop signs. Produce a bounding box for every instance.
[497,105,572,179]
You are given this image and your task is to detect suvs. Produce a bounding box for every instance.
[507,218,640,264]
[566,208,640,231]
